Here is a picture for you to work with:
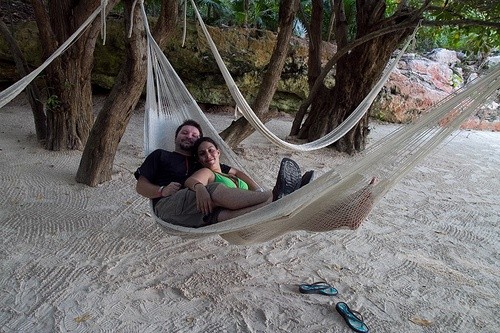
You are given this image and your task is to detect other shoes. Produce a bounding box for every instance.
[301,170,315,187]
[272,158,302,202]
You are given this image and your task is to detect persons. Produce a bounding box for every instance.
[183,137,376,245]
[134,119,314,228]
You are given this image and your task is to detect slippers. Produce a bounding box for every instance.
[336,301,369,333]
[299,281,338,296]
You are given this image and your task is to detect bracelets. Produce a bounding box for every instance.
[194,183,203,189]
[160,186,166,197]
